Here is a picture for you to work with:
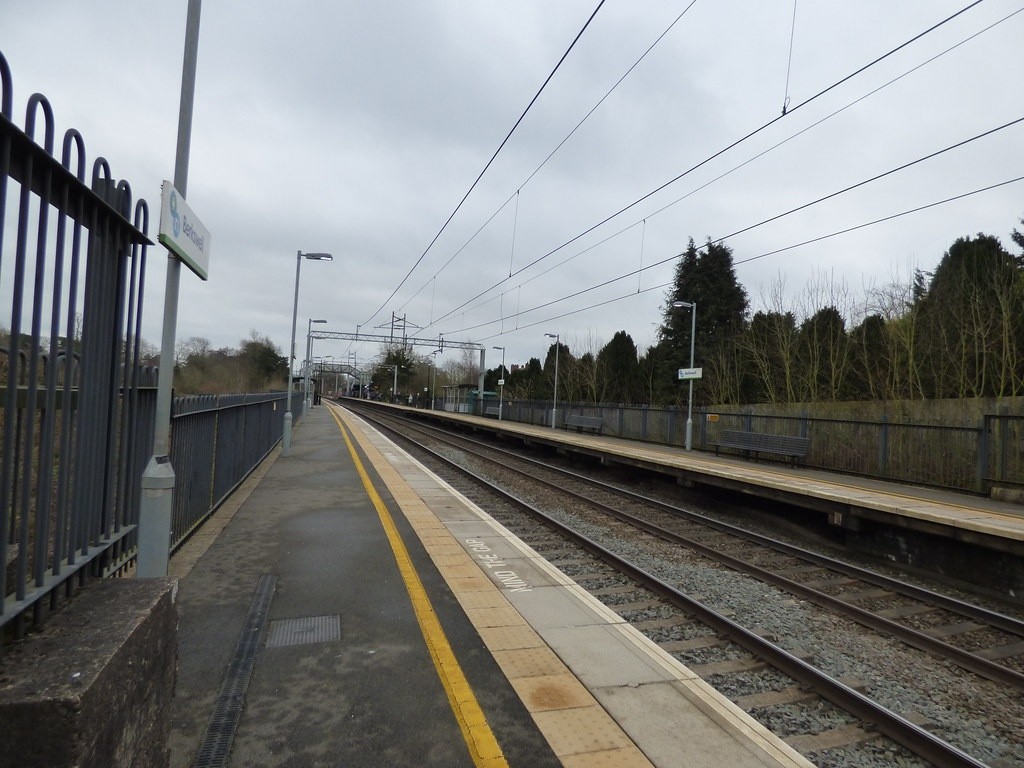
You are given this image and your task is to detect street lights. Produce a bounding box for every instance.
[319,355,331,405]
[493,346,506,421]
[302,319,328,422]
[400,365,409,406]
[543,333,559,430]
[672,302,695,452]
[308,336,325,405]
[279,251,332,458]
[425,362,432,410]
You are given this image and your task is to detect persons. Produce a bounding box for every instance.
[396,392,401,405]
[409,393,413,407]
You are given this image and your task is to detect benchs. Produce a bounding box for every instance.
[706,430,810,468]
[480,407,499,418]
[561,415,604,436]
